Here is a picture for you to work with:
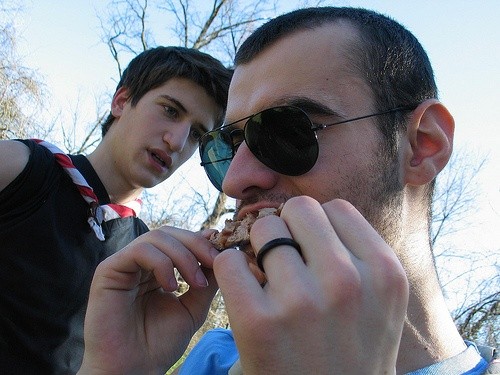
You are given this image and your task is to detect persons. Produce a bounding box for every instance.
[0,46,236,374]
[74,6,499,374]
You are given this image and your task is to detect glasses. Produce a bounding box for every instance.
[198,104,412,193]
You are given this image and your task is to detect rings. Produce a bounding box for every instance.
[257,237,300,273]
